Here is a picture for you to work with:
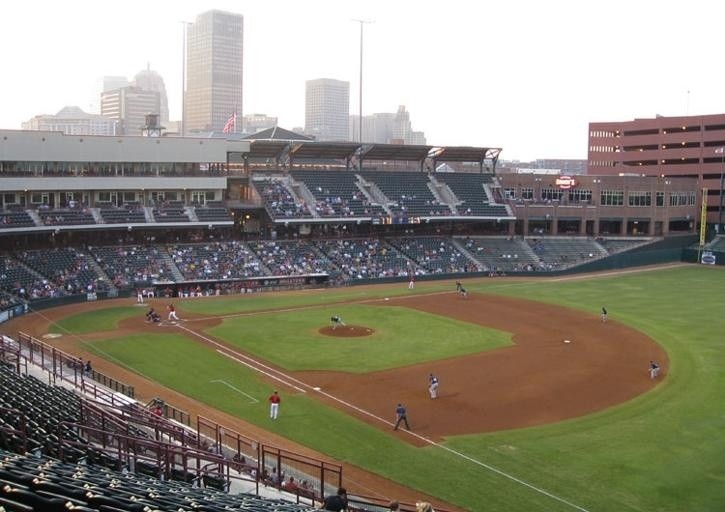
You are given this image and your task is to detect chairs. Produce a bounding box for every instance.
[0,169,649,512]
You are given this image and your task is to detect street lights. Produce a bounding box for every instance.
[176,21,190,135]
[352,13,371,166]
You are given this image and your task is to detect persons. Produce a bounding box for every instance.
[388,499,401,512]
[317,184,609,280]
[145,308,161,322]
[329,314,346,330]
[165,302,180,321]
[416,502,436,512]
[337,486,349,512]
[392,403,411,431]
[312,495,343,512]
[0,169,321,309]
[600,306,608,323]
[147,405,323,501]
[456,280,467,296]
[409,276,416,289]
[267,390,281,419]
[83,360,93,373]
[73,356,83,368]
[427,373,439,399]
[648,360,662,378]
